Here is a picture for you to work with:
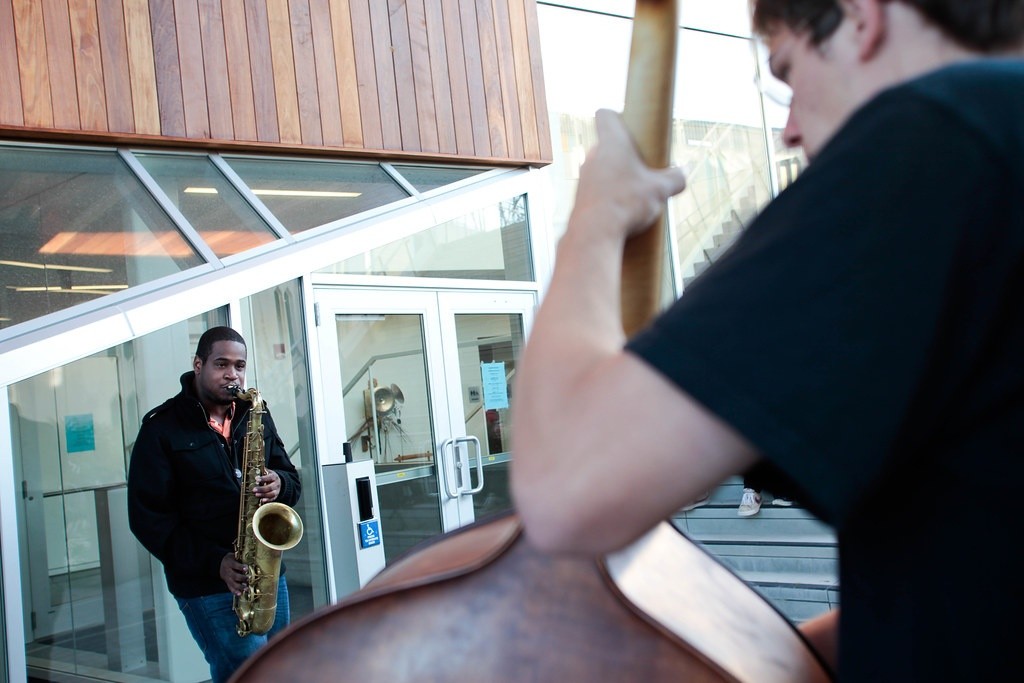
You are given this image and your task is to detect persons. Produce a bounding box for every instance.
[508,0,1023,682]
[128,326,302,683]
[733,471,795,518]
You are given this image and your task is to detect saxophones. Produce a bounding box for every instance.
[227,384,305,637]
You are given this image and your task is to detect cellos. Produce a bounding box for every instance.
[229,2,828,682]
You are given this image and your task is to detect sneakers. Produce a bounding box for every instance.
[771,495,793,507]
[738,488,763,517]
[679,489,710,511]
